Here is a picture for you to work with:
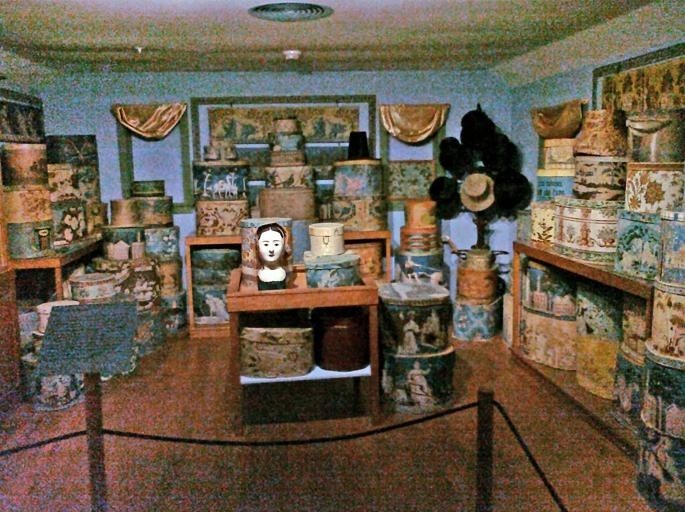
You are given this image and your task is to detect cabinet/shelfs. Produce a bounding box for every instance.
[184,231,392,340]
[226,266,382,432]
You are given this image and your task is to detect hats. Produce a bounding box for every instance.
[430,110,528,219]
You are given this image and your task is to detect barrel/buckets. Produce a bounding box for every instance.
[0,115,685,512]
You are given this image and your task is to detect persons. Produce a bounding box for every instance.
[255,220,289,290]
[576,300,597,336]
[420,308,445,352]
[404,256,425,282]
[406,359,440,412]
[402,309,422,353]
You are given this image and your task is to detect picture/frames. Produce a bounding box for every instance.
[191,95,377,163]
[381,104,446,211]
[593,43,684,111]
[117,107,194,214]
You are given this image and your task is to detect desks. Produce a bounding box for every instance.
[513,241,654,463]
[13,240,104,301]
